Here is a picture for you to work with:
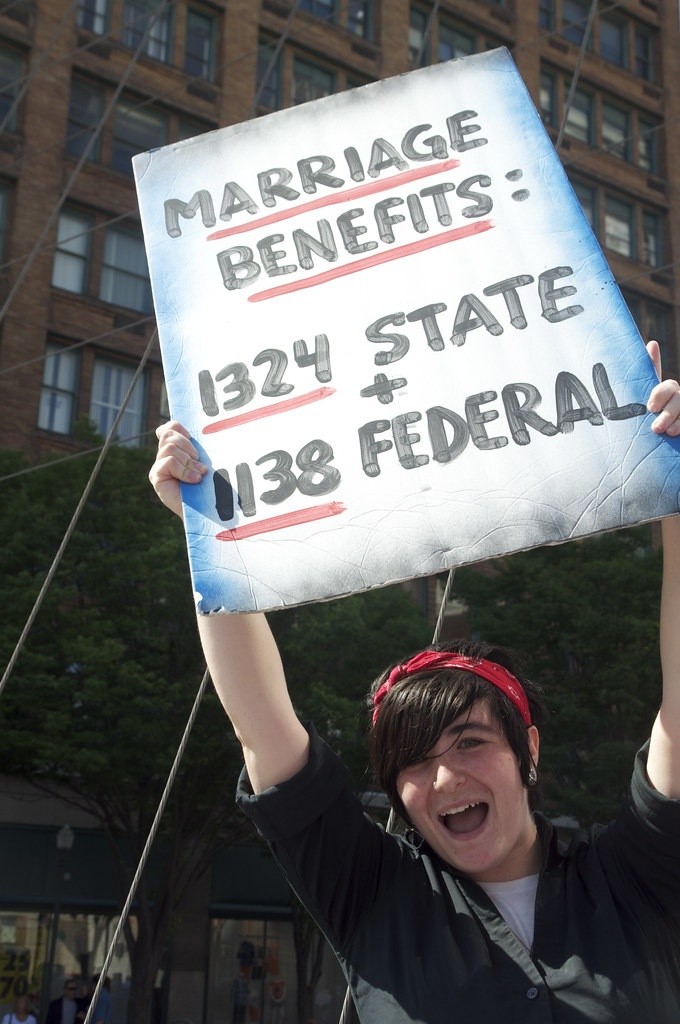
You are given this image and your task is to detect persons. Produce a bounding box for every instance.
[236,936,255,973]
[91,973,112,1023]
[80,983,90,998]
[229,971,252,1023]
[45,979,89,1023]
[1,992,40,1023]
[150,340,680,1024]
[269,976,288,1023]
